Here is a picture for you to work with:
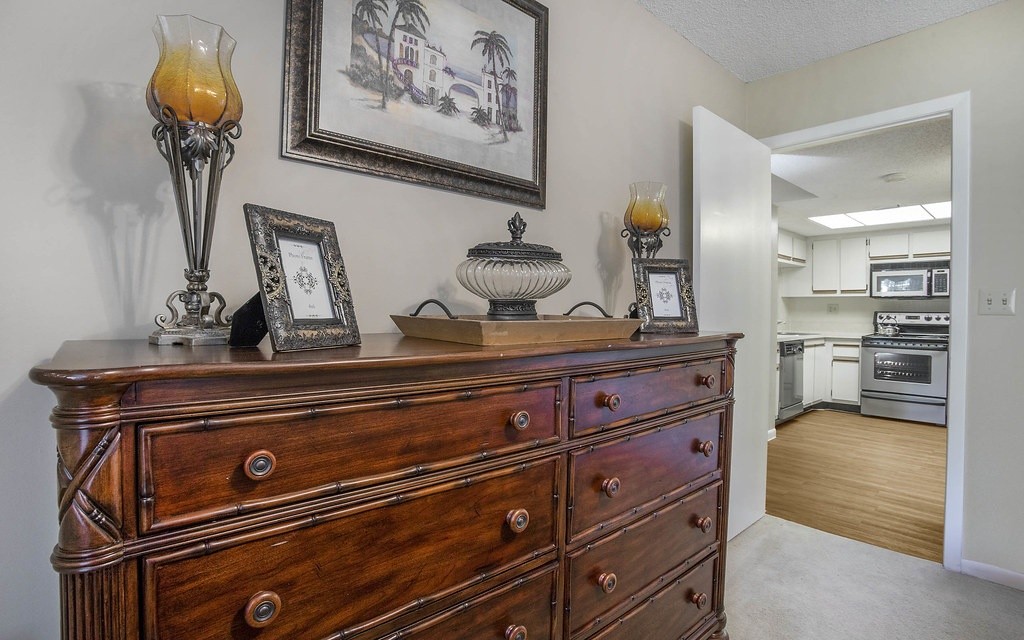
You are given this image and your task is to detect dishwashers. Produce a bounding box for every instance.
[779,341,804,420]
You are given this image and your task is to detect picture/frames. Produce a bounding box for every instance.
[228,203,361,351]
[629,258,698,333]
[282,0,548,209]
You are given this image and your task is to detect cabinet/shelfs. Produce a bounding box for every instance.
[777,229,807,267]
[31,332,746,640]
[804,339,826,404]
[870,230,950,257]
[812,237,867,292]
[831,343,860,401]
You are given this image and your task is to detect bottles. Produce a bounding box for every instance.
[455,211,572,320]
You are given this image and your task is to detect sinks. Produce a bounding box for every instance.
[777,331,822,335]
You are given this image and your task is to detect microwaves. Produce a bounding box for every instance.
[870,261,950,300]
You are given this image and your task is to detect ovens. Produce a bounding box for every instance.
[860,347,948,427]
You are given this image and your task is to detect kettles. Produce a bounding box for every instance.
[874,317,900,336]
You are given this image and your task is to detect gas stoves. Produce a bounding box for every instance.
[861,310,950,349]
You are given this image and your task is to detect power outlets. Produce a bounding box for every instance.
[828,305,839,314]
[978,288,1015,315]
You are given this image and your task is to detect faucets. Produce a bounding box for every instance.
[777,320,787,325]
